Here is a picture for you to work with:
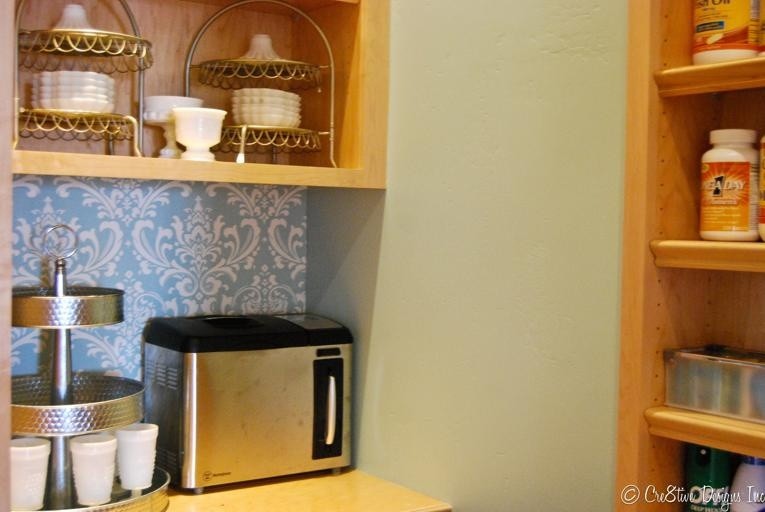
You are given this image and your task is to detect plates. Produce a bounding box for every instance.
[31,70,114,112]
[231,86,304,129]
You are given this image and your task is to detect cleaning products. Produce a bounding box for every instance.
[683,444,765,512]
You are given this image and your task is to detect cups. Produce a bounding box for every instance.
[8,436,51,512]
[240,33,281,61]
[52,3,94,31]
[68,431,117,507]
[117,421,159,489]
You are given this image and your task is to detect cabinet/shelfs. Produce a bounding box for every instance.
[0,0,392,191]
[613,0,765,512]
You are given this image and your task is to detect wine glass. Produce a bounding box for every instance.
[141,95,205,156]
[170,106,227,160]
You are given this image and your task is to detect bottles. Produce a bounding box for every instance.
[681,442,765,511]
[697,128,765,243]
[690,1,760,66]
